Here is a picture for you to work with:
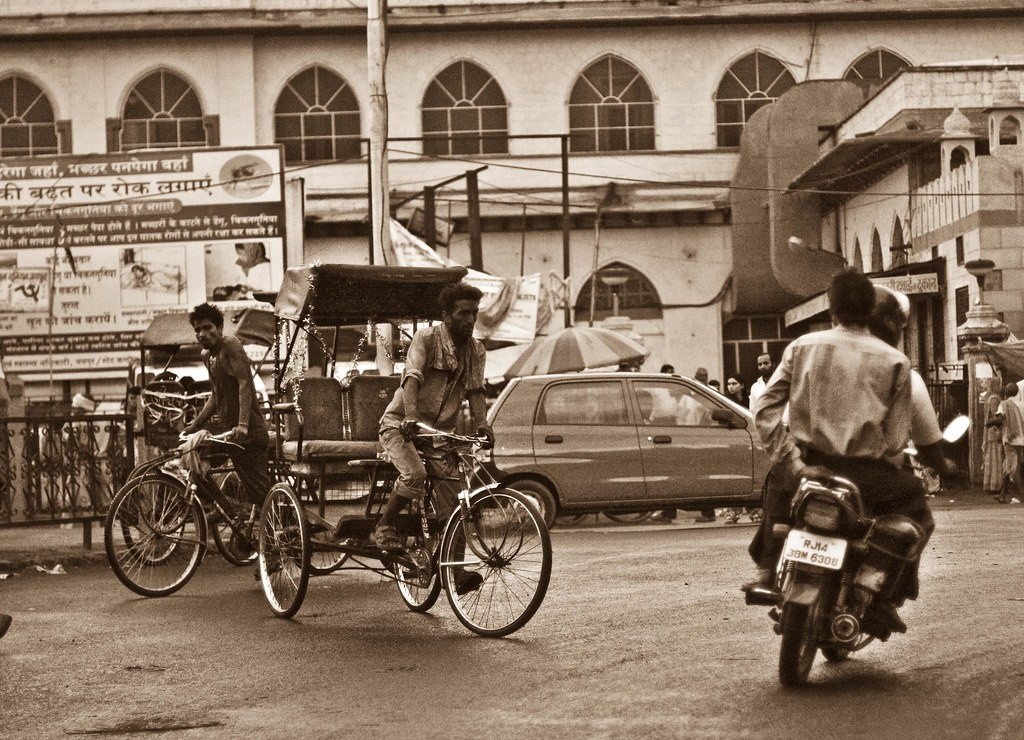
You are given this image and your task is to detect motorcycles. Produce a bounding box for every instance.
[738,473,927,690]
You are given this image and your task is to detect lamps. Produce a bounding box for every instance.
[789,236,848,267]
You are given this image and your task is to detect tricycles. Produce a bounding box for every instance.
[103,261,553,640]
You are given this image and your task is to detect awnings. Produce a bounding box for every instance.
[780,135,933,205]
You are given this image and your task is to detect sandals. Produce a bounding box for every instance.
[442,570,482,592]
[370,524,402,552]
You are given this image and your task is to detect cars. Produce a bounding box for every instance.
[473,370,793,532]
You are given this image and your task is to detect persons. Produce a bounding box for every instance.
[738,267,956,634]
[981,377,1024,503]
[234,242,271,292]
[152,356,271,431]
[185,303,284,580]
[619,354,790,524]
[60,393,142,529]
[370,282,495,592]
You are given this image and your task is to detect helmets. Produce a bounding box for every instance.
[868,284,910,348]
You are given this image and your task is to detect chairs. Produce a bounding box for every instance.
[636,389,655,426]
[147,380,186,434]
[347,375,401,459]
[281,377,347,460]
[559,392,620,424]
[186,381,213,427]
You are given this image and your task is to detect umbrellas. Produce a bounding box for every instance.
[504,329,650,379]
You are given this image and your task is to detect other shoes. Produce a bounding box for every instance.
[740,566,777,593]
[207,504,236,524]
[993,495,1005,503]
[695,515,716,523]
[749,509,763,523]
[875,599,907,634]
[253,554,283,580]
[652,515,672,524]
[724,513,739,524]
